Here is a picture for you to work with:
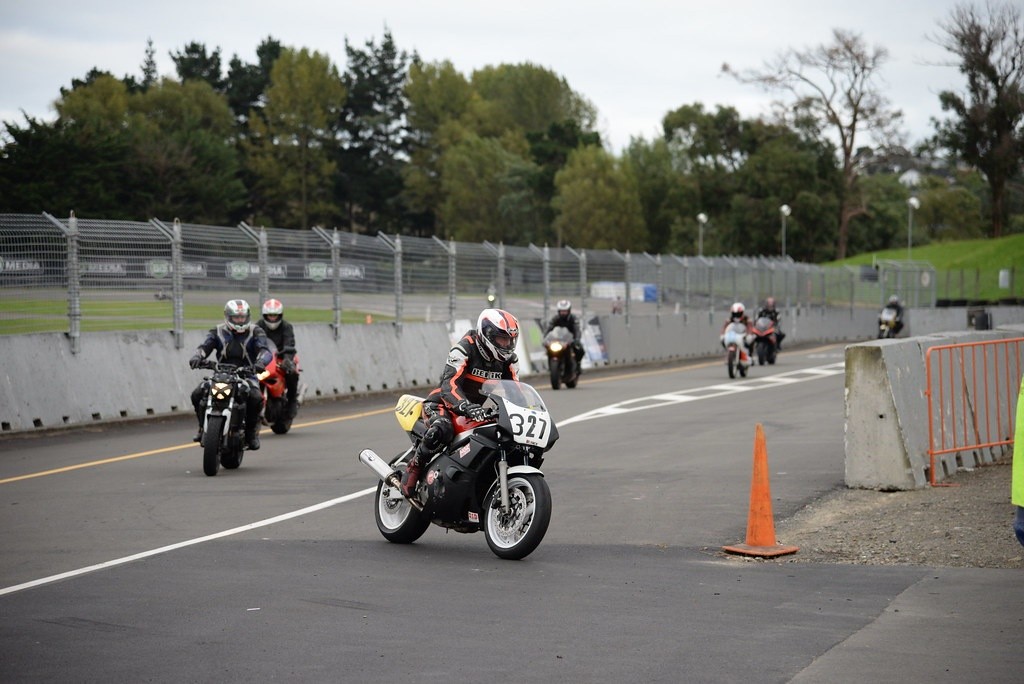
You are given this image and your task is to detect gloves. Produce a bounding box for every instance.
[189,347,206,369]
[459,401,487,422]
[253,349,272,374]
[281,355,295,375]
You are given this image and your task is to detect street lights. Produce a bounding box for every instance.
[906,198,920,259]
[780,204,791,256]
[697,212,706,255]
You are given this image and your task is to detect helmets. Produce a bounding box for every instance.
[261,299,283,330]
[764,298,776,312]
[474,308,520,363]
[889,296,897,306]
[224,299,251,336]
[556,300,571,317]
[731,303,745,322]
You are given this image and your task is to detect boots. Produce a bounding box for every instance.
[244,416,260,450]
[193,414,204,441]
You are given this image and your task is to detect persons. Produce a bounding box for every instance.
[720,302,754,356]
[402,309,529,498]
[882,295,903,333]
[188,299,273,450]
[758,298,786,349]
[256,299,299,418]
[544,299,585,374]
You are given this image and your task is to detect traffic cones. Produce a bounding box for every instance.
[723,425,798,558]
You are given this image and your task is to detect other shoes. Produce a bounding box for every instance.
[287,399,297,419]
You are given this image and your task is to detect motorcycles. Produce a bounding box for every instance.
[542,327,579,389]
[260,338,308,434]
[189,359,254,475]
[878,309,897,339]
[721,323,757,378]
[358,376,559,561]
[751,317,778,366]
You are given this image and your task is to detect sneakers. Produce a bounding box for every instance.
[401,457,421,498]
